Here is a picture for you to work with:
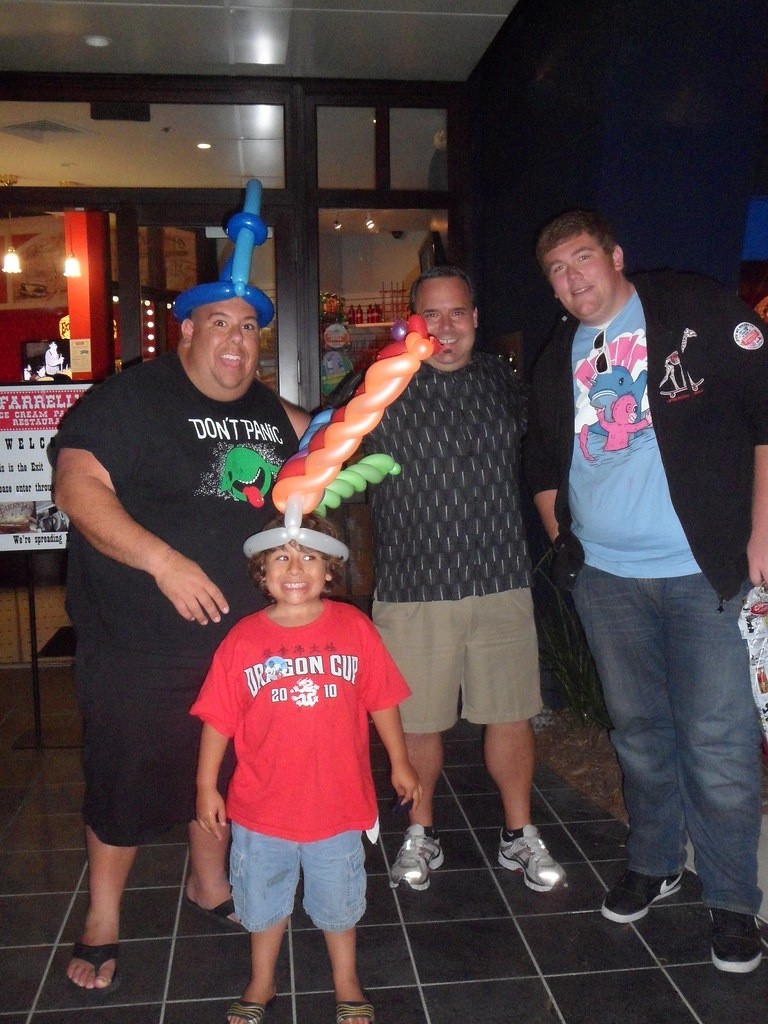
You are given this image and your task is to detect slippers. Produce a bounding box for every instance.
[226,994,277,1024]
[68,931,122,1000]
[184,896,249,932]
[335,992,375,1024]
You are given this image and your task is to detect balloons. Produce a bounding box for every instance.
[243,313,443,568]
[174,175,275,329]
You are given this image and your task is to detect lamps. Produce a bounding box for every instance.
[63,216,81,277]
[332,219,342,231]
[365,212,375,229]
[1,212,22,274]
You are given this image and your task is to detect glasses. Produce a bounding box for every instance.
[592,328,613,374]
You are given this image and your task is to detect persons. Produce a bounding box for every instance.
[311,267,570,895]
[50,277,319,992]
[517,211,768,977]
[190,511,426,1024]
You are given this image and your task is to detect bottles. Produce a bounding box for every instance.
[319,292,382,324]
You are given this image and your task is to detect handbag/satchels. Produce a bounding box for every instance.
[738,581,768,740]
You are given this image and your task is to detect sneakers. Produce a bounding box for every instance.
[709,907,762,973]
[601,869,682,923]
[498,824,568,892]
[389,824,444,891]
